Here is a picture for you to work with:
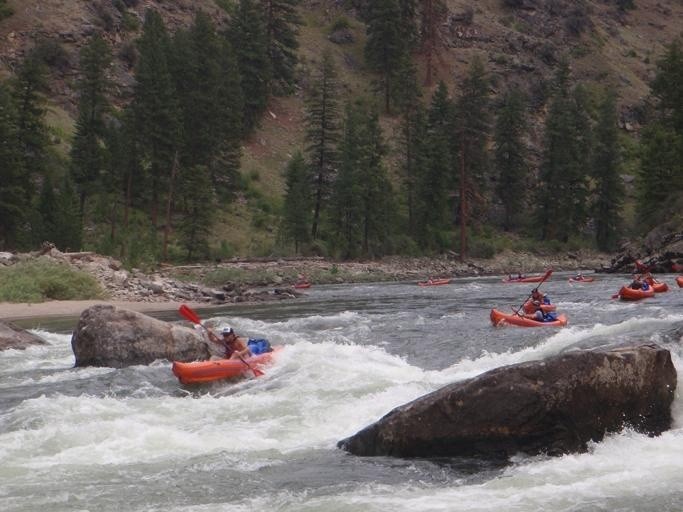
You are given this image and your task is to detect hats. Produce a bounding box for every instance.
[532,300,540,305]
[218,327,233,334]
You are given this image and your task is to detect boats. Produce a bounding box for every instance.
[490,308,567,329]
[171,347,280,386]
[650,281,667,293]
[502,276,549,283]
[417,279,449,287]
[676,276,682,288]
[293,283,311,289]
[618,284,654,299]
[568,277,592,284]
[521,300,557,314]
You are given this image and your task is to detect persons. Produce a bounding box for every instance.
[531,288,548,304]
[644,272,656,284]
[427,274,436,284]
[632,274,644,290]
[207,327,252,361]
[523,300,545,322]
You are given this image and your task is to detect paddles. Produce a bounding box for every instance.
[179,304,264,377]
[513,270,552,314]
[612,264,656,299]
